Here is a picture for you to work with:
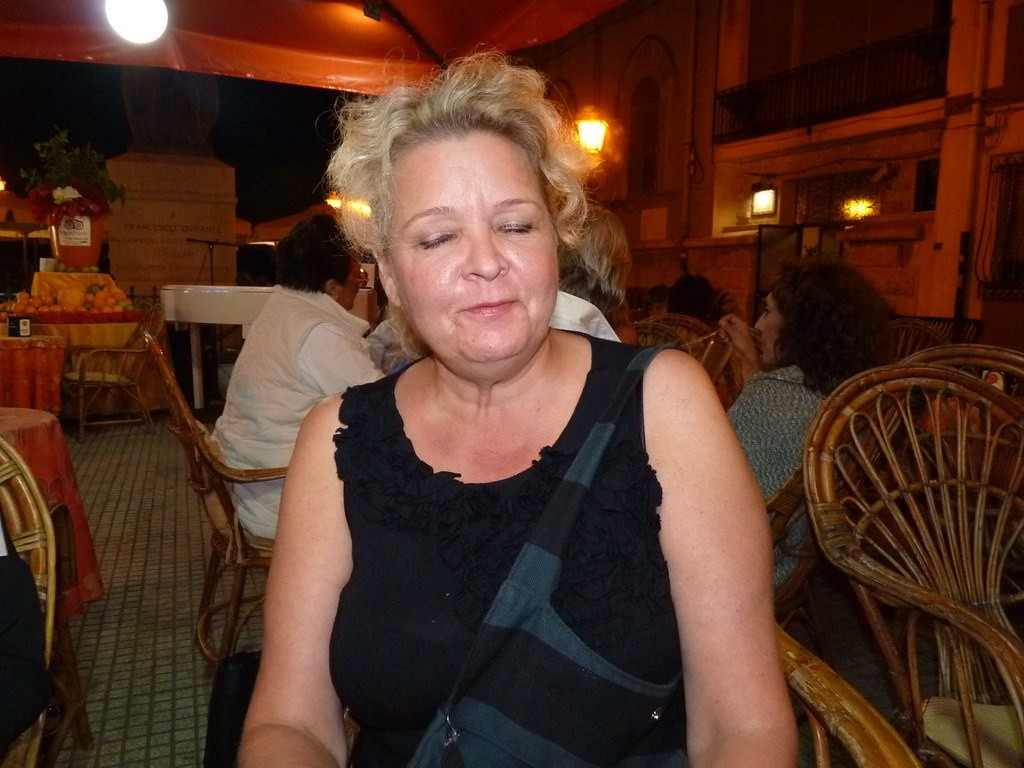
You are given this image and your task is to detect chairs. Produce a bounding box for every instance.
[0,304,1024,768]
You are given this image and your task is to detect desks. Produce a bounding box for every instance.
[0,334,64,416]
[0,407,104,618]
[0,310,172,420]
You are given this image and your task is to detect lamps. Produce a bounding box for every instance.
[574,112,609,152]
[751,186,778,216]
[362,3,381,22]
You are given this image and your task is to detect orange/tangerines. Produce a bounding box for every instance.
[55,261,100,273]
[0,282,136,314]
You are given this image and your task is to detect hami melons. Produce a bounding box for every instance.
[57,287,85,306]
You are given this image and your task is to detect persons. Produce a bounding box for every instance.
[213,214,388,555]
[235,52,800,768]
[723,255,890,623]
[646,272,719,329]
[547,194,640,345]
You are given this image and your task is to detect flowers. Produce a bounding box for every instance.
[51,186,84,205]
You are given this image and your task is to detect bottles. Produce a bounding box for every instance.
[20,316,30,336]
[98,231,110,273]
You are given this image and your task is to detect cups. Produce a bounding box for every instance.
[39,258,56,272]
[8,316,20,337]
[700,334,733,383]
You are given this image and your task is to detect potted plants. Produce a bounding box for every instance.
[14,124,126,273]
